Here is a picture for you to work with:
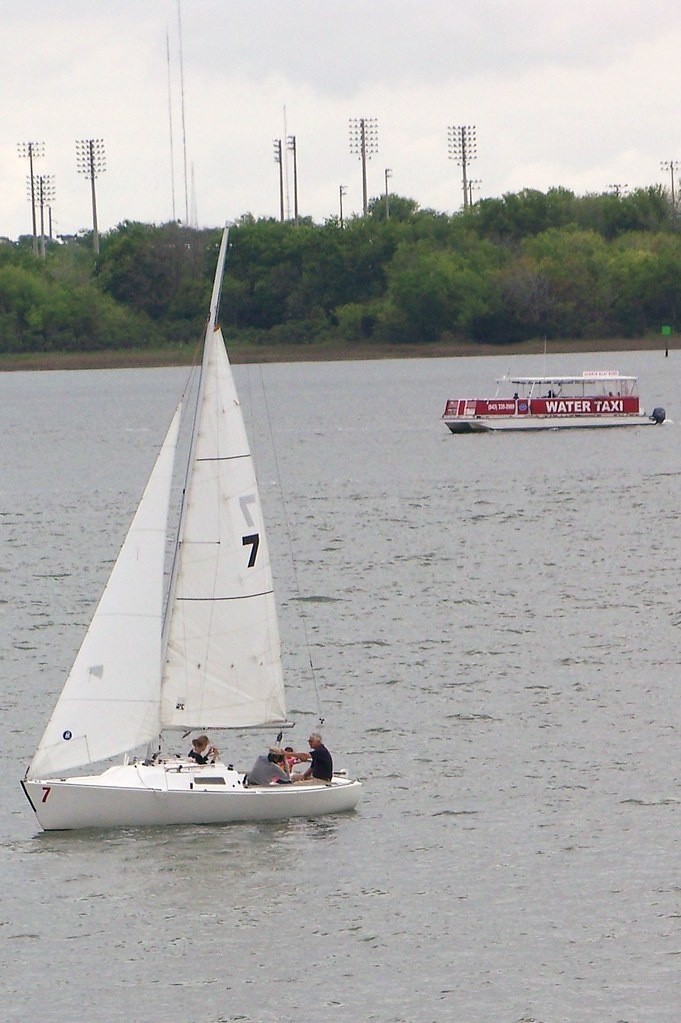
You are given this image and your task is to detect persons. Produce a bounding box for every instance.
[247,734,332,786]
[513,393,519,399]
[609,392,613,397]
[547,390,556,398]
[188,736,218,765]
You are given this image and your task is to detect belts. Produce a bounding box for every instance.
[313,776,328,781]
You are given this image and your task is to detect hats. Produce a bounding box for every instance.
[269,747,283,756]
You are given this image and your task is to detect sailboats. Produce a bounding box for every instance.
[19,229,360,832]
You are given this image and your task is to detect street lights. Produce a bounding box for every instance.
[461,180,483,207]
[385,169,392,219]
[349,118,378,216]
[17,141,45,257]
[660,161,681,206]
[26,175,57,260]
[274,138,284,221]
[447,125,477,209]
[75,138,107,254]
[340,186,347,228]
[609,184,628,197]
[287,136,298,220]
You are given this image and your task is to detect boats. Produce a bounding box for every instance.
[440,370,666,434]
